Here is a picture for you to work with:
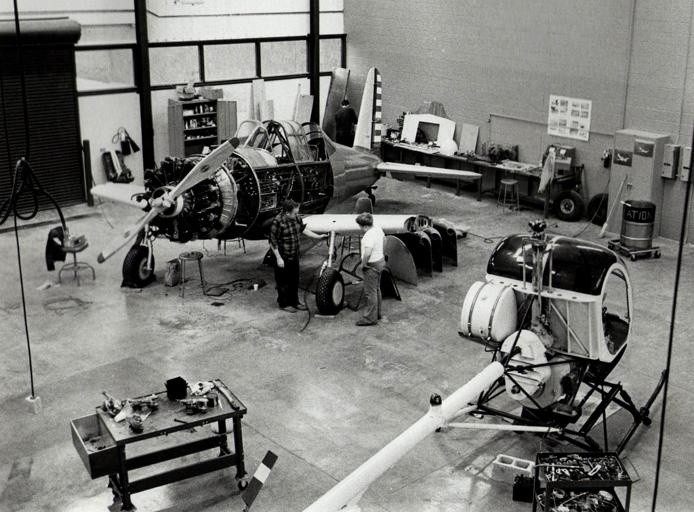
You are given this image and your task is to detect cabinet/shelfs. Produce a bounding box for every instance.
[532,445,634,512]
[166,94,238,158]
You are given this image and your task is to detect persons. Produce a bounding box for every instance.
[354,212,387,325]
[334,100,356,148]
[269,199,329,314]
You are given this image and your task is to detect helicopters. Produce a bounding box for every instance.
[272,207,678,511]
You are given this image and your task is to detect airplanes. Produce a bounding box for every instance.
[88,66,481,307]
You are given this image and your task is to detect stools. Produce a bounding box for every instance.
[177,250,206,298]
[57,242,96,290]
[496,177,521,214]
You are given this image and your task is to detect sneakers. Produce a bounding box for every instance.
[355,315,382,326]
[280,302,307,313]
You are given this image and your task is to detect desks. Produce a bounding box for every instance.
[381,133,580,218]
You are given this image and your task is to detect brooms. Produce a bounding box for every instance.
[597,175,627,238]
